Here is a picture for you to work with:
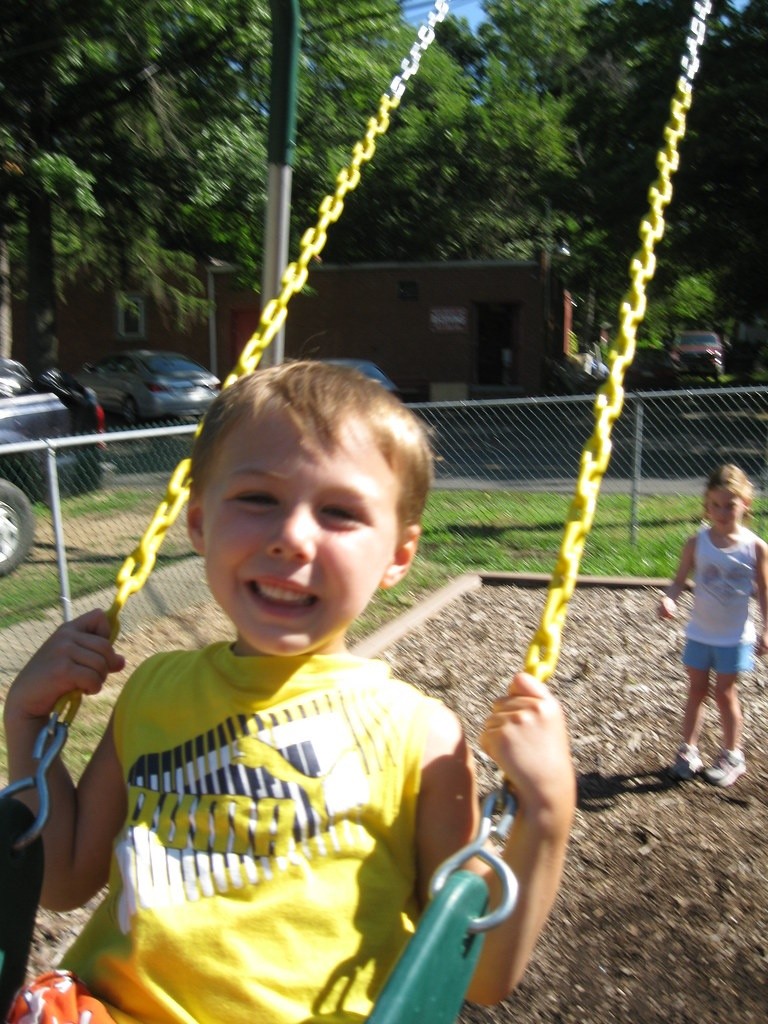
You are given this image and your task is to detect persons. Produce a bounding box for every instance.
[1,363,576,1024]
[658,464,768,788]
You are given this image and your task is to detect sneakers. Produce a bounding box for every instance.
[669,743,703,779]
[703,749,746,787]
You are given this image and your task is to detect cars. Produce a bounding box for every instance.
[660,330,723,382]
[0,359,107,576]
[321,359,405,402]
[73,348,222,428]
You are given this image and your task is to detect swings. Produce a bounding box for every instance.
[0,0,711,1024]
